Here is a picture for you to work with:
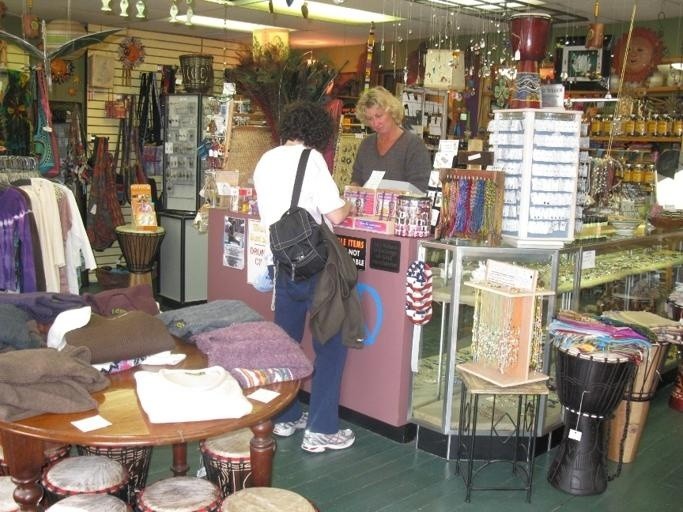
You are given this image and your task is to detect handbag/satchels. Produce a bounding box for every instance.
[33,128,60,178]
[141,141,165,177]
[269,208,328,283]
[115,164,159,207]
[86,188,125,252]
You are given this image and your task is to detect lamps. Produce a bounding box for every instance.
[251,27,289,65]
[584,1,604,50]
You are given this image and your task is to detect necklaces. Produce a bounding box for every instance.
[436,175,499,242]
[380,0,626,82]
[411,280,561,420]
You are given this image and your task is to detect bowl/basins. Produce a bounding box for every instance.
[649,216,682,230]
[609,221,642,237]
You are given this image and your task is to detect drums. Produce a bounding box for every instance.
[137,476,222,512]
[76,446,151,506]
[46,494,133,512]
[115,225,166,299]
[509,12,553,109]
[199,426,276,497]
[218,487,319,511]
[606,343,671,464]
[41,454,128,505]
[548,344,634,496]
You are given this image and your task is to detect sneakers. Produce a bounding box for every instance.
[300,428,355,454]
[273,413,309,436]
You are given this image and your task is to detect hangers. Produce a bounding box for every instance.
[0,156,40,185]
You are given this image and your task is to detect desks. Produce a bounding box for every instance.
[0,336,302,512]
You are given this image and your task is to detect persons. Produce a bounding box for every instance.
[252,101,357,453]
[347,84,434,195]
[226,220,241,245]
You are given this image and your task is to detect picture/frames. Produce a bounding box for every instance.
[555,31,613,91]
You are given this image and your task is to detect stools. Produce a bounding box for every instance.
[449,368,551,503]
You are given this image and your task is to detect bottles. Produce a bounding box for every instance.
[587,113,683,187]
[241,196,249,214]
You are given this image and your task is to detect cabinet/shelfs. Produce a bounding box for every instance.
[407,218,683,461]
[335,96,368,137]
[562,87,683,191]
